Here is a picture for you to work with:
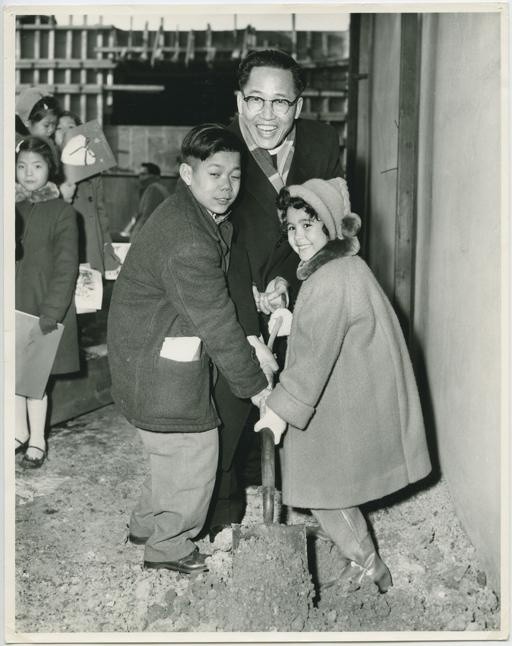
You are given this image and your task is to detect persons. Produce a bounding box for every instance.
[128,161,171,244]
[53,110,122,274]
[251,175,435,597]
[15,136,82,471]
[15,87,62,180]
[193,48,347,554]
[103,120,275,578]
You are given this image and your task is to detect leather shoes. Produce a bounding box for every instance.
[129,533,149,545]
[19,438,48,468]
[143,547,212,574]
[15,436,29,454]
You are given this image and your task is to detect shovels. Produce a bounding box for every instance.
[232,292,308,571]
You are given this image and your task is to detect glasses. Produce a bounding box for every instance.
[241,89,303,114]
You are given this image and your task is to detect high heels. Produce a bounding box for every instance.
[337,534,393,594]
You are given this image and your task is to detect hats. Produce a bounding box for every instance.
[15,88,52,121]
[286,177,351,241]
